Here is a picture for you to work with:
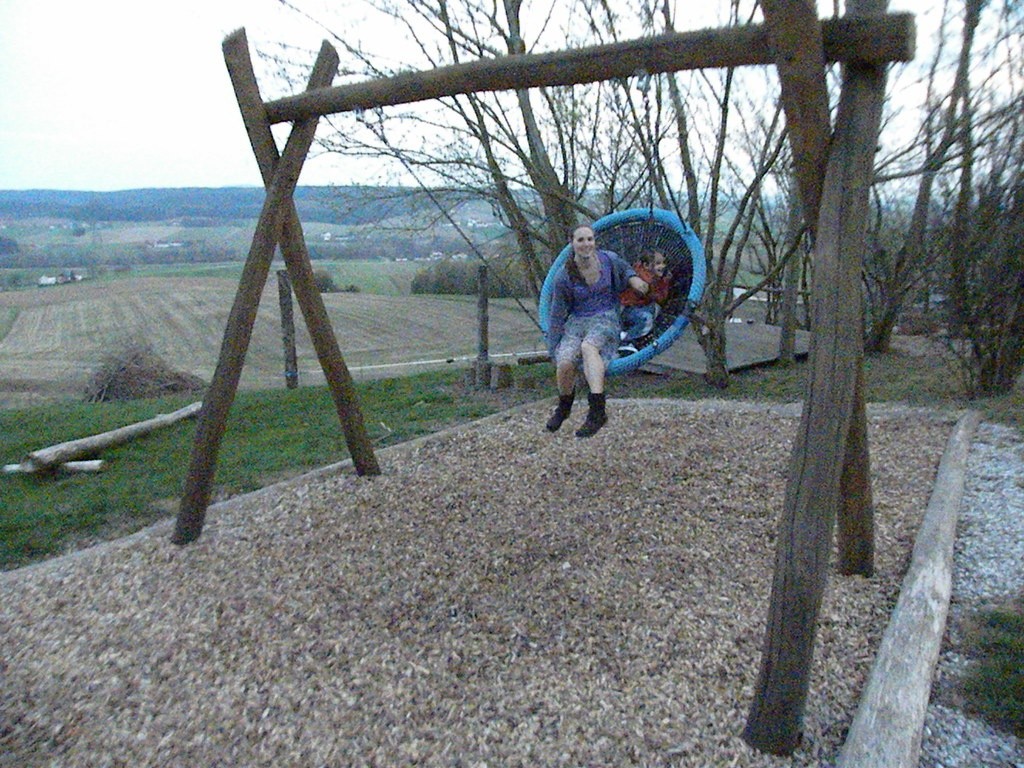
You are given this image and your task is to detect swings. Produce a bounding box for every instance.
[350,74,708,378]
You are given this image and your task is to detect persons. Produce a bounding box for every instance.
[617,247,673,354]
[547,225,649,437]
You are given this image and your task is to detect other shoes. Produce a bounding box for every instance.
[617,342,638,355]
[619,330,628,341]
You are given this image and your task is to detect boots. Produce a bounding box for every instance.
[545,385,577,432]
[576,390,609,437]
